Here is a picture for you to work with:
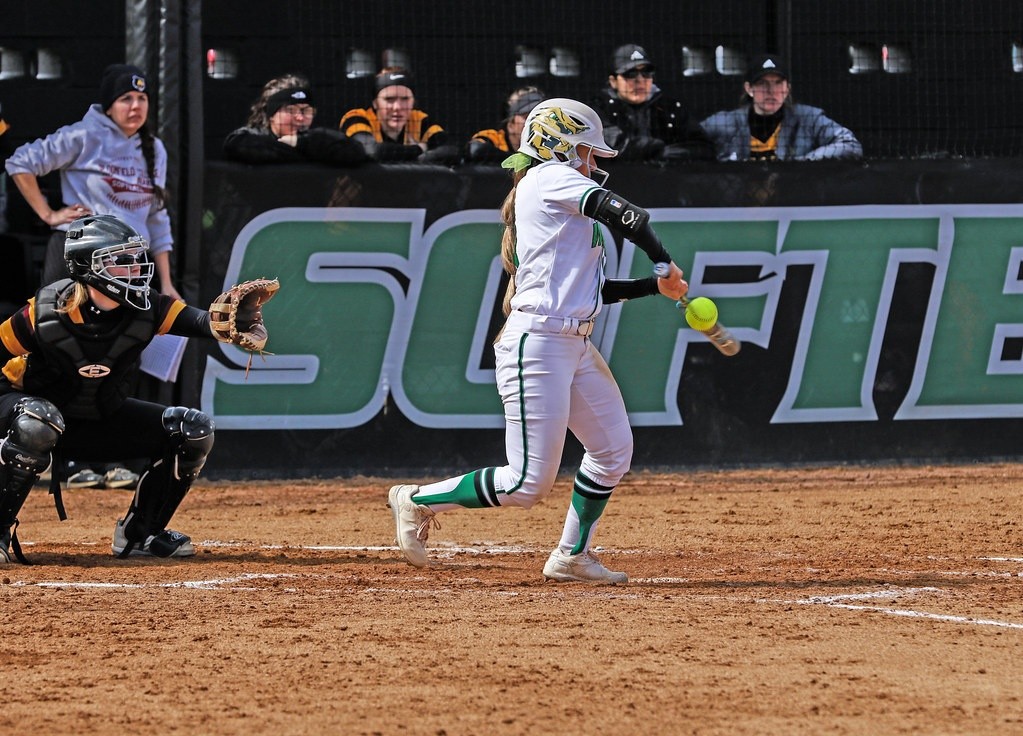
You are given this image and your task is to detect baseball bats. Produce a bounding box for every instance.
[653,261,741,357]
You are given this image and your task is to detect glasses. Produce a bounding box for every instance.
[621,66,654,78]
[103,254,141,266]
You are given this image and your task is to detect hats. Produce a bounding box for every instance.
[101,63,149,113]
[614,45,651,74]
[748,54,791,82]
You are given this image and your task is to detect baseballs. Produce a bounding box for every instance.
[686,296,718,331]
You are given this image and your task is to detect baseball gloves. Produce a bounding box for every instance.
[210,279,280,352]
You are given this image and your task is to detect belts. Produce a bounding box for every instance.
[546,318,594,335]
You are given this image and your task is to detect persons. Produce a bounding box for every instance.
[5,64,183,488]
[0,213,280,562]
[339,66,462,165]
[389,98,688,583]
[466,85,548,164]
[699,54,862,160]
[589,44,711,158]
[224,74,365,166]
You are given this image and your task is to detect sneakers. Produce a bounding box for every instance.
[0,529,13,563]
[67,469,104,488]
[543,547,627,583]
[111,520,195,557]
[388,484,442,569]
[103,468,139,487]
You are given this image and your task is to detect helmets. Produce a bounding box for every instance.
[64,214,152,314]
[518,99,618,187]
